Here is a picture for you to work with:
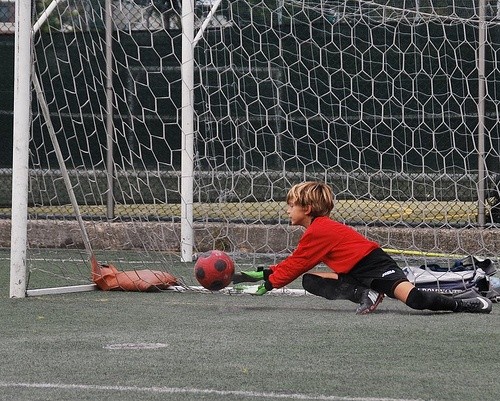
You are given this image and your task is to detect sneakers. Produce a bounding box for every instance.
[355,288,387,316]
[453,296,492,314]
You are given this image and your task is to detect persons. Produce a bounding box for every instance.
[230,181,492,313]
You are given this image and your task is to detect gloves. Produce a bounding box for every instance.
[256,281,273,296]
[231,267,273,284]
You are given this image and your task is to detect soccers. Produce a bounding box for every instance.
[194,250,234,291]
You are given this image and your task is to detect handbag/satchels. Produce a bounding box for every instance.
[402,255,500,303]
